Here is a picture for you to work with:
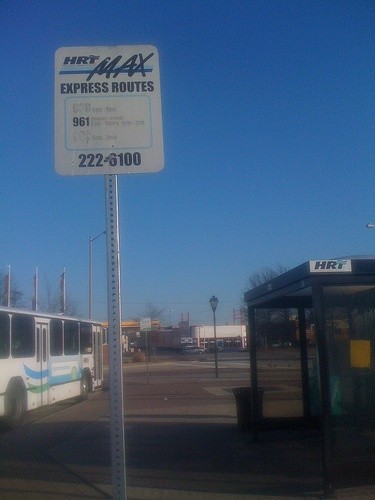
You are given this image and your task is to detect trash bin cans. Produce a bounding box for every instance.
[355,368,375,415]
[330,371,352,413]
[232,384,266,431]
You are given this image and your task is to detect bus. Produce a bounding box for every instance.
[0,307,107,421]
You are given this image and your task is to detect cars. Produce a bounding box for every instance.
[178,345,205,355]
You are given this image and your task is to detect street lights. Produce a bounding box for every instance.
[209,296,220,377]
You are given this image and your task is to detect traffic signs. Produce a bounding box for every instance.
[53,45,167,176]
